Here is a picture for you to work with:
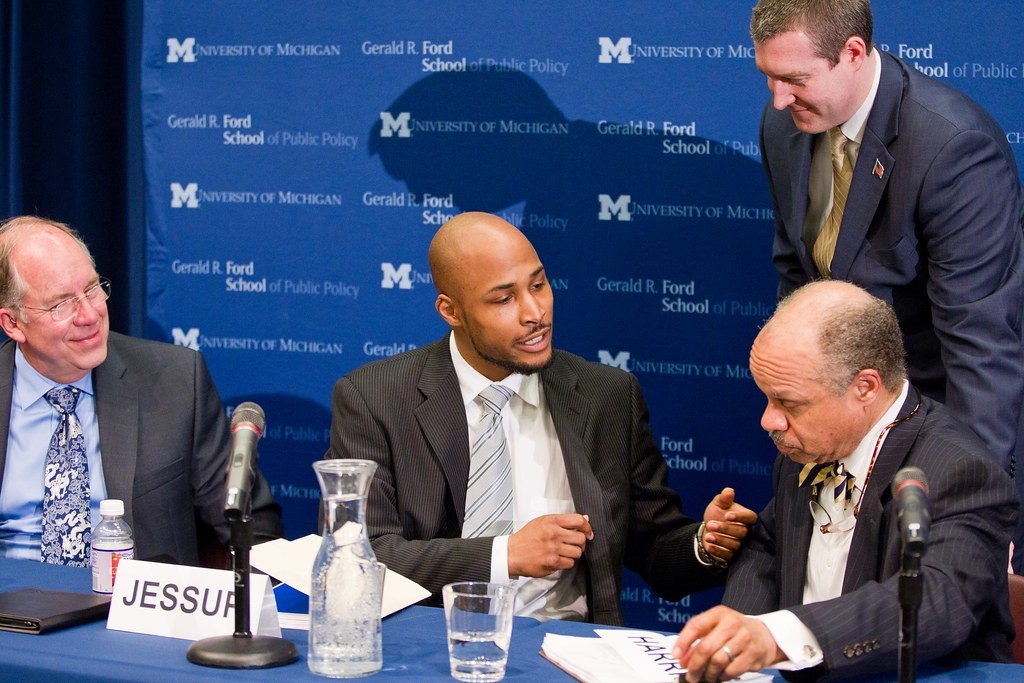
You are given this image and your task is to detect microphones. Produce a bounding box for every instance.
[891,465,932,557]
[222,401,265,524]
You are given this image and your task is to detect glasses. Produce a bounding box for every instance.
[807,498,858,534]
[16,276,111,324]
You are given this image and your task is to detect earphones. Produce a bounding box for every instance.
[820,525,831,534]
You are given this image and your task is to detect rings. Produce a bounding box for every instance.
[722,646,733,662]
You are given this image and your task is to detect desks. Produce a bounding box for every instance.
[0,553,1024,683]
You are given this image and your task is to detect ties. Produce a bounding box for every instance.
[813,124,856,283]
[458,383,514,539]
[42,384,93,568]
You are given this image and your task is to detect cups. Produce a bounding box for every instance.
[441,581,518,683]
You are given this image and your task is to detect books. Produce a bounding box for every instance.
[1,588,112,634]
[537,629,775,683]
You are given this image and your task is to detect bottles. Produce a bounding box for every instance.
[305,458,388,679]
[89,498,136,595]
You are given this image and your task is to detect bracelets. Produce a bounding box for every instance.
[697,521,728,569]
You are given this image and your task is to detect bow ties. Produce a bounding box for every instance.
[796,458,858,510]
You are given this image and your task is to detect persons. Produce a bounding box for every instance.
[671,275,1024,683]
[751,1,1024,577]
[0,216,284,570]
[318,212,758,627]
